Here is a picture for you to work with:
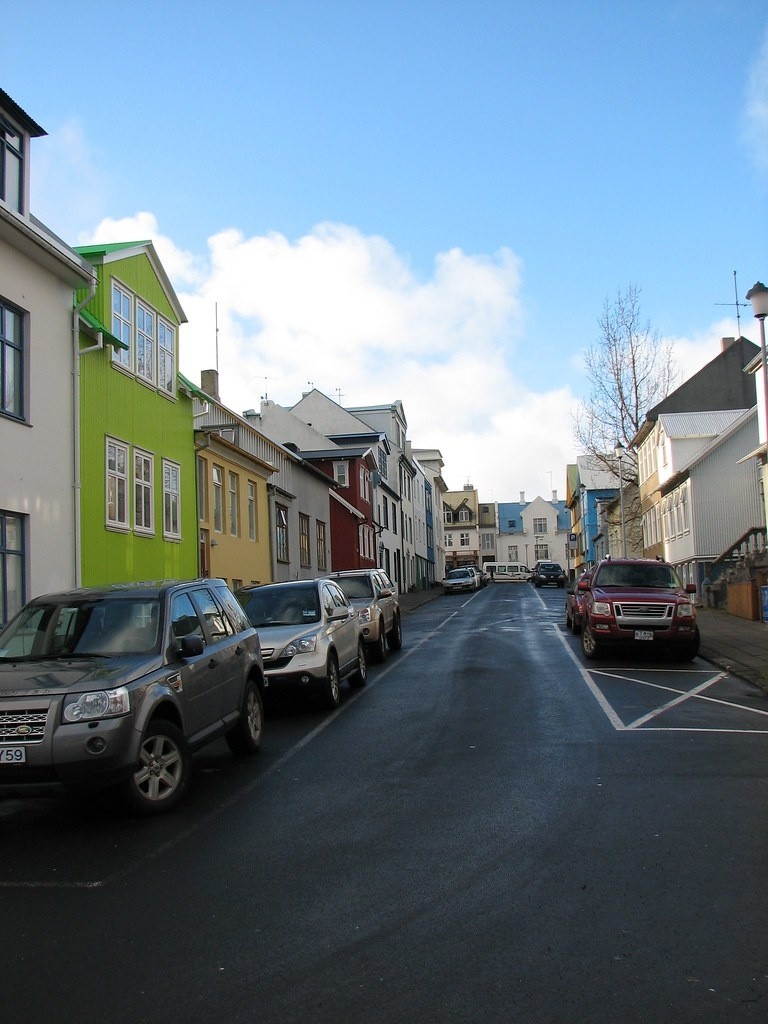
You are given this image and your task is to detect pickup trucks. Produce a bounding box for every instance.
[315,569,404,667]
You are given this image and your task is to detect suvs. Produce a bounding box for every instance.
[578,554,702,663]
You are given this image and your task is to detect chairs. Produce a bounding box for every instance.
[463,573,467,577]
[90,605,134,650]
[520,568,523,572]
[141,604,178,649]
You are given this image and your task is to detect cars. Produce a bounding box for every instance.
[532,561,552,585]
[229,578,369,712]
[534,563,566,589]
[0,580,268,818]
[565,572,592,635]
[442,564,488,595]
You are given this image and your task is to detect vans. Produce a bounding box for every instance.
[483,562,534,583]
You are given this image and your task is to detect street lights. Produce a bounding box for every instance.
[614,441,627,558]
[744,280,767,416]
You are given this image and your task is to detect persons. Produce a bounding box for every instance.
[490,568,493,579]
[535,563,540,571]
